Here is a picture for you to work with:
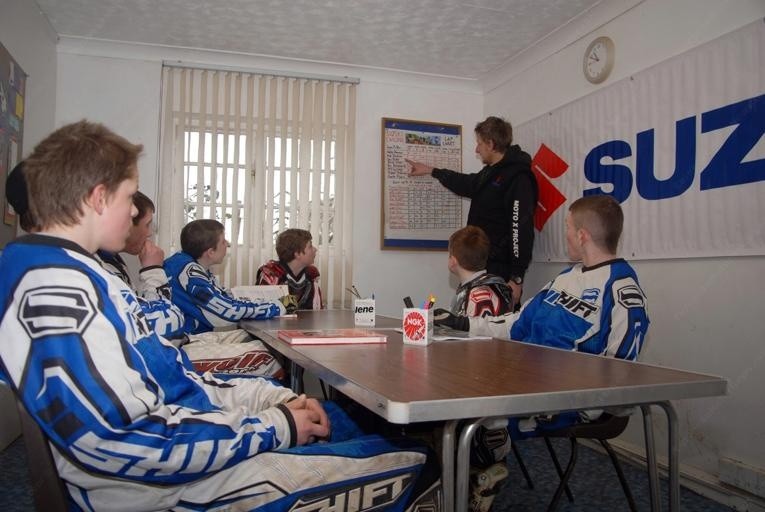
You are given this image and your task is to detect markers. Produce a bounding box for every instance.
[403,296,414,308]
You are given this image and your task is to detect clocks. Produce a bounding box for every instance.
[582,36,614,84]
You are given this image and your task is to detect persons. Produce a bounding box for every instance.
[0,119,445,511]
[405,116,539,312]
[433,225,514,332]
[5,158,281,378]
[116,190,286,382]
[255,230,324,309]
[468,194,649,511]
[161,219,300,333]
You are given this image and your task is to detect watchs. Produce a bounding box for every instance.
[509,275,523,285]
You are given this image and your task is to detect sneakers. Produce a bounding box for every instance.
[455,422,514,512]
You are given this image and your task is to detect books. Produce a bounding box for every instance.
[230,284,299,318]
[278,328,387,345]
[394,322,492,341]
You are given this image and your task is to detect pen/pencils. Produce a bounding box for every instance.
[279,286,285,297]
[345,285,374,300]
[419,294,436,309]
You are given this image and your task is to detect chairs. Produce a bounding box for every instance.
[12,396,71,512]
[510,413,640,512]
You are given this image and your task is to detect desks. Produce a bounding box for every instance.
[240,309,726,512]
